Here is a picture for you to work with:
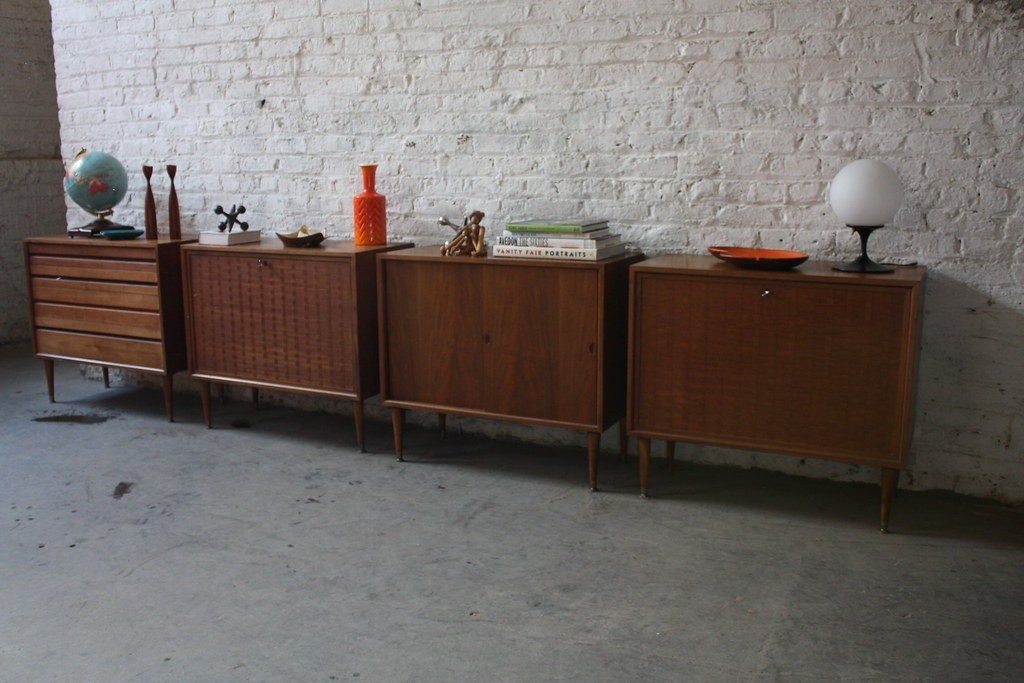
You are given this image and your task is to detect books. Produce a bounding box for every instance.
[493,215,626,260]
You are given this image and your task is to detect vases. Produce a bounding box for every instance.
[352,164,387,246]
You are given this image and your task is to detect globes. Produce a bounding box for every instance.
[64,148,136,239]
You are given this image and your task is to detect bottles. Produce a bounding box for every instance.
[352,165,388,246]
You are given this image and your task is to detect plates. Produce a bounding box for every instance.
[276,224,328,248]
[706,246,809,271]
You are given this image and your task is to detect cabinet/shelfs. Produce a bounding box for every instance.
[179,236,415,455]
[374,246,648,494]
[626,254,927,533]
[22,232,202,422]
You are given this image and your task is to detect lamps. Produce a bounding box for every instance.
[829,158,904,275]
